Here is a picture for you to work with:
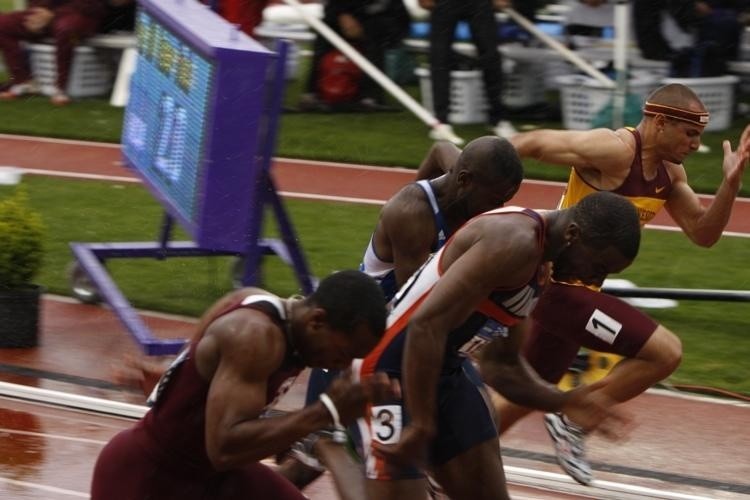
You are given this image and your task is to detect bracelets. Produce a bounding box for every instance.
[317,391,343,427]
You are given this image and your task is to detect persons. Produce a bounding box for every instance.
[78,267,404,500]
[1,0,750,156]
[277,132,528,500]
[492,82,750,487]
[292,187,646,498]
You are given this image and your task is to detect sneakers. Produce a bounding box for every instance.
[544,411,593,486]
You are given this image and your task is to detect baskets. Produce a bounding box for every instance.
[24,43,114,98]
[499,47,571,107]
[552,72,660,131]
[414,68,492,124]
[660,75,739,132]
